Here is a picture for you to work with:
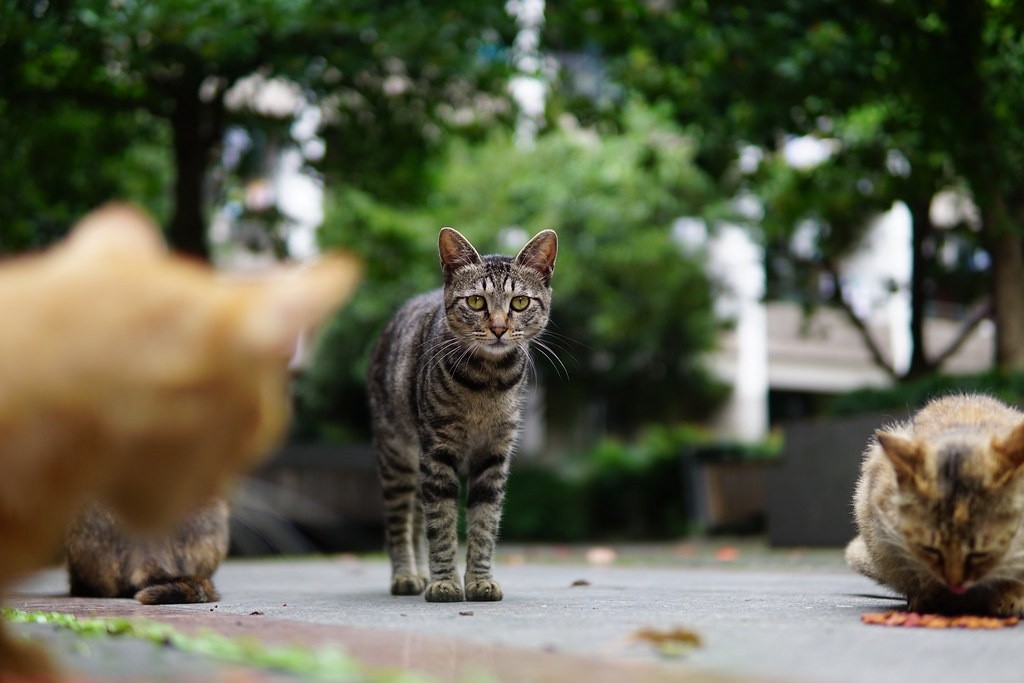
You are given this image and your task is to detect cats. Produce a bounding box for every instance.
[0,199,363,683]
[843,388,1024,618]
[363,227,558,601]
